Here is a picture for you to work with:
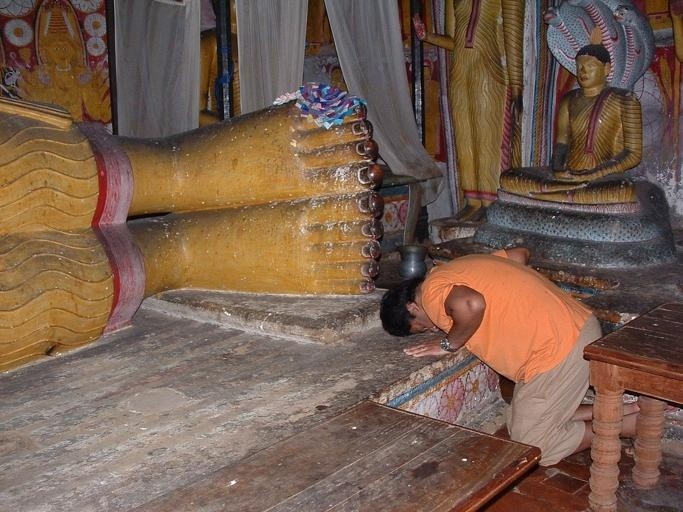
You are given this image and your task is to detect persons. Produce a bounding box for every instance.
[552,43,646,191]
[409,1,525,224]
[0,82,383,373]
[380,243,639,469]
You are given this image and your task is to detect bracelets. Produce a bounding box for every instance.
[438,336,459,354]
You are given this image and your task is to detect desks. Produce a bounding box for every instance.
[585,295,683,511]
[128,397,549,510]
